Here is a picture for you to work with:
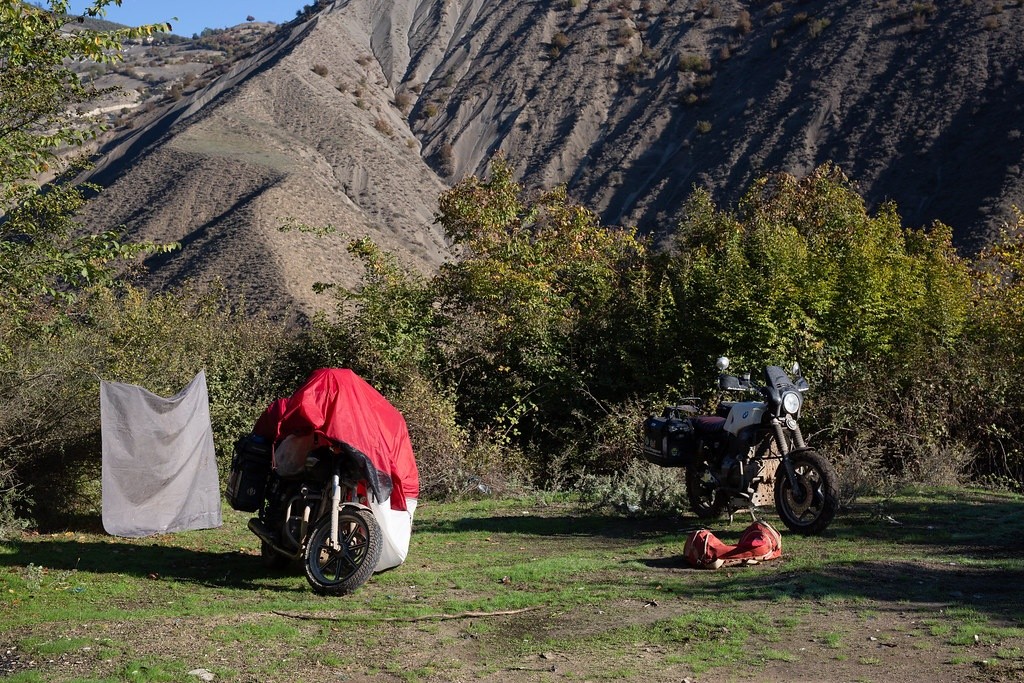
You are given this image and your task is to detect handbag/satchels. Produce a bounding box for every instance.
[684,519,781,570]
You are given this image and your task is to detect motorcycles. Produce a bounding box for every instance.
[641,355,840,532]
[225,367,409,596]
